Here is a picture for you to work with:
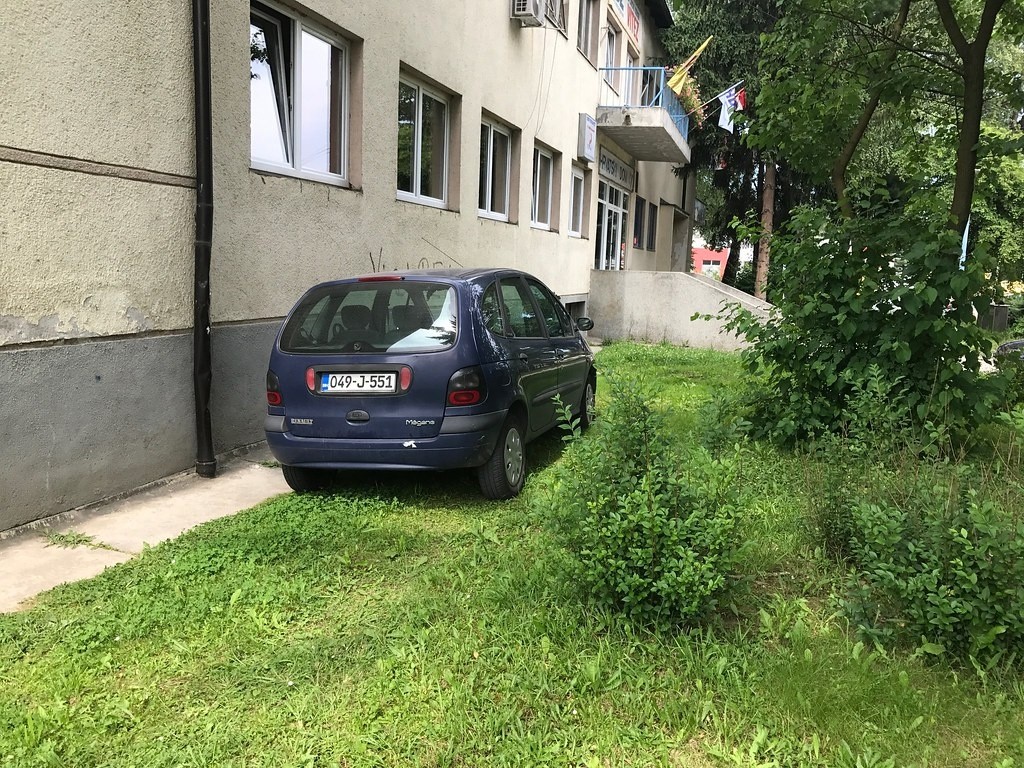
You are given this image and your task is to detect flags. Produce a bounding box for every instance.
[667,35,714,96]
[718,84,739,134]
[734,90,745,112]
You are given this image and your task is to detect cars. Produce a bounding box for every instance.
[264,268,599,501]
[996,340,1024,363]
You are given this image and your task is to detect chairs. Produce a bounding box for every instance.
[385,305,422,346]
[330,305,370,347]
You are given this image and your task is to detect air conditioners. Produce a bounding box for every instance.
[514,0,540,25]
